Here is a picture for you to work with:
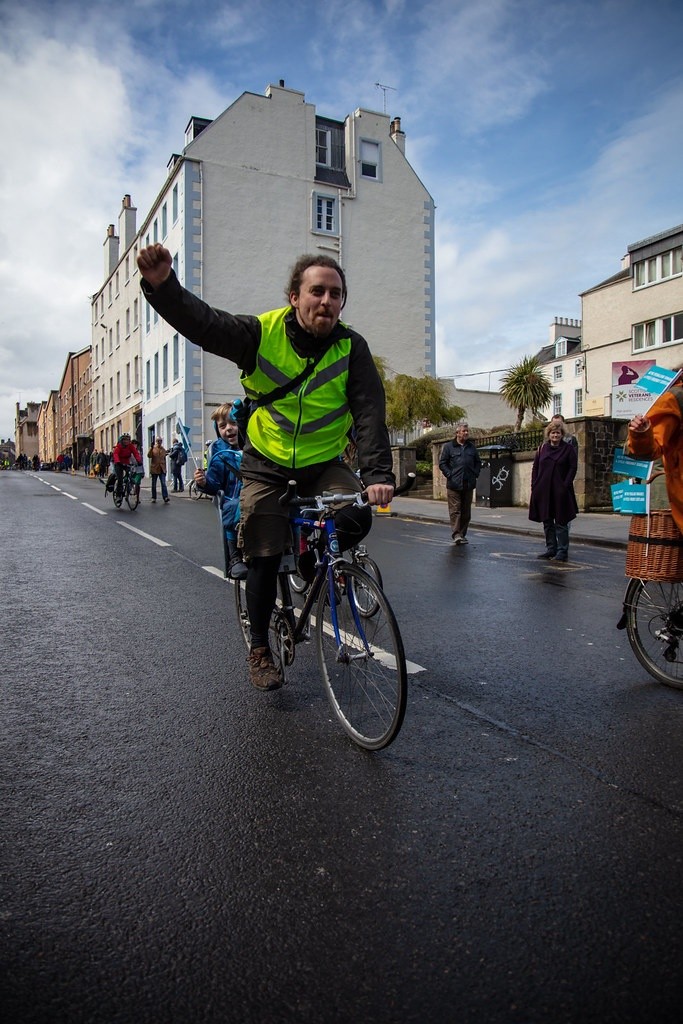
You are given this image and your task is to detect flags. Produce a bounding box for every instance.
[175,422,191,453]
[634,365,676,395]
[613,449,650,480]
[620,484,646,513]
[611,479,629,511]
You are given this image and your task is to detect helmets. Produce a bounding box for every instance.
[119,432,131,440]
[205,440,212,446]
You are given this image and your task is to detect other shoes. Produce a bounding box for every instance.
[115,497,122,503]
[231,559,248,578]
[456,538,469,543]
[170,489,184,493]
[151,498,155,502]
[164,498,169,502]
[537,552,551,559]
[551,556,567,562]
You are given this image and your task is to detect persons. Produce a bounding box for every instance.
[131,440,144,483]
[147,437,170,503]
[300,441,359,587]
[551,415,578,531]
[16,453,40,472]
[528,420,579,562]
[169,439,185,493]
[113,433,141,503]
[81,446,115,478]
[137,243,396,691]
[203,440,212,469]
[438,421,480,543]
[623,379,683,534]
[57,453,72,471]
[194,400,248,579]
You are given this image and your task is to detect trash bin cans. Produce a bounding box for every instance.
[476,445,514,508]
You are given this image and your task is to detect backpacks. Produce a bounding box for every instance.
[176,445,187,466]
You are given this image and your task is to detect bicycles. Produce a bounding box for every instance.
[185,477,216,501]
[105,463,143,512]
[234,472,419,755]
[615,579,682,689]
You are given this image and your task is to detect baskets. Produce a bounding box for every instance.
[624,509,683,582]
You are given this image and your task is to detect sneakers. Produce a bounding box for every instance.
[245,647,281,692]
[296,549,341,606]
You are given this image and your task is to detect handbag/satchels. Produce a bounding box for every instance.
[106,474,115,492]
